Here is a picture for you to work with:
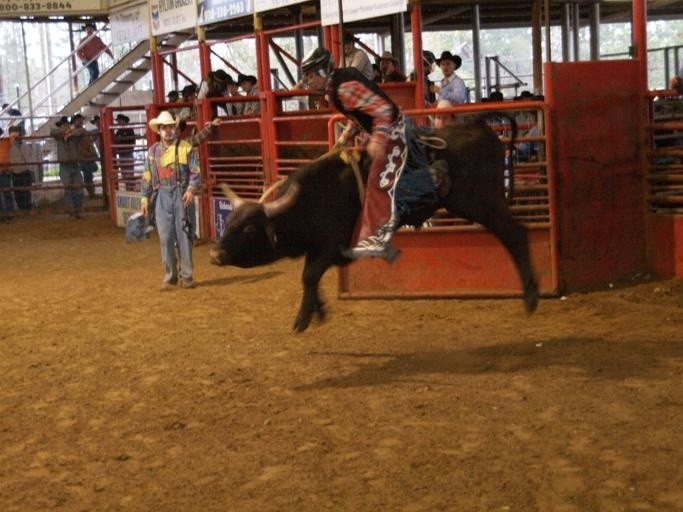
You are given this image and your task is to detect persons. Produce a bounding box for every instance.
[50,115,85,214]
[173,114,222,249]
[1,103,25,146]
[114,114,135,191]
[90,114,100,151]
[338,32,374,81]
[166,63,261,122]
[140,109,203,291]
[374,52,405,81]
[0,129,14,222]
[78,20,113,85]
[301,46,407,252]
[511,91,535,138]
[7,126,35,209]
[516,95,543,155]
[477,91,513,149]
[371,63,382,85]
[69,113,100,198]
[431,49,466,103]
[426,102,454,128]
[294,76,329,110]
[411,50,437,99]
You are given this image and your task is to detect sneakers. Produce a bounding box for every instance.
[157,276,193,292]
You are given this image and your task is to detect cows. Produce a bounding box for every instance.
[209,109,541,336]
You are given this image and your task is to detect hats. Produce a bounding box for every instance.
[167,69,257,98]
[341,32,461,70]
[481,90,544,102]
[149,111,180,136]
[54,112,131,128]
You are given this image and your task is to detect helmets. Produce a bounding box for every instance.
[303,46,332,74]
